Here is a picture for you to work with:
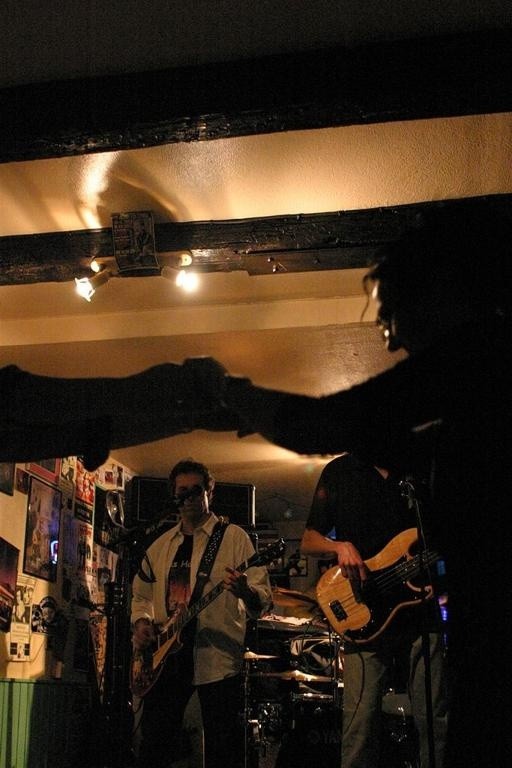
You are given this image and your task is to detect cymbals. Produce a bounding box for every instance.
[245,652,279,661]
[249,670,335,685]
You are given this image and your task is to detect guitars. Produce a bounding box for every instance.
[316,527,443,644]
[129,535,288,699]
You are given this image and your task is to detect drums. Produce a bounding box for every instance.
[275,718,343,768]
[377,664,412,717]
[289,691,335,731]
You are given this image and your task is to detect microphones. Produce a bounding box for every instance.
[172,483,203,500]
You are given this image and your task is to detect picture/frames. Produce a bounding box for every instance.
[22,456,64,584]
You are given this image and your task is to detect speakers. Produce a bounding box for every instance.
[405,474,418,510]
[124,476,257,528]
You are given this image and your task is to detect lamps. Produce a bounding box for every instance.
[71,264,200,302]
